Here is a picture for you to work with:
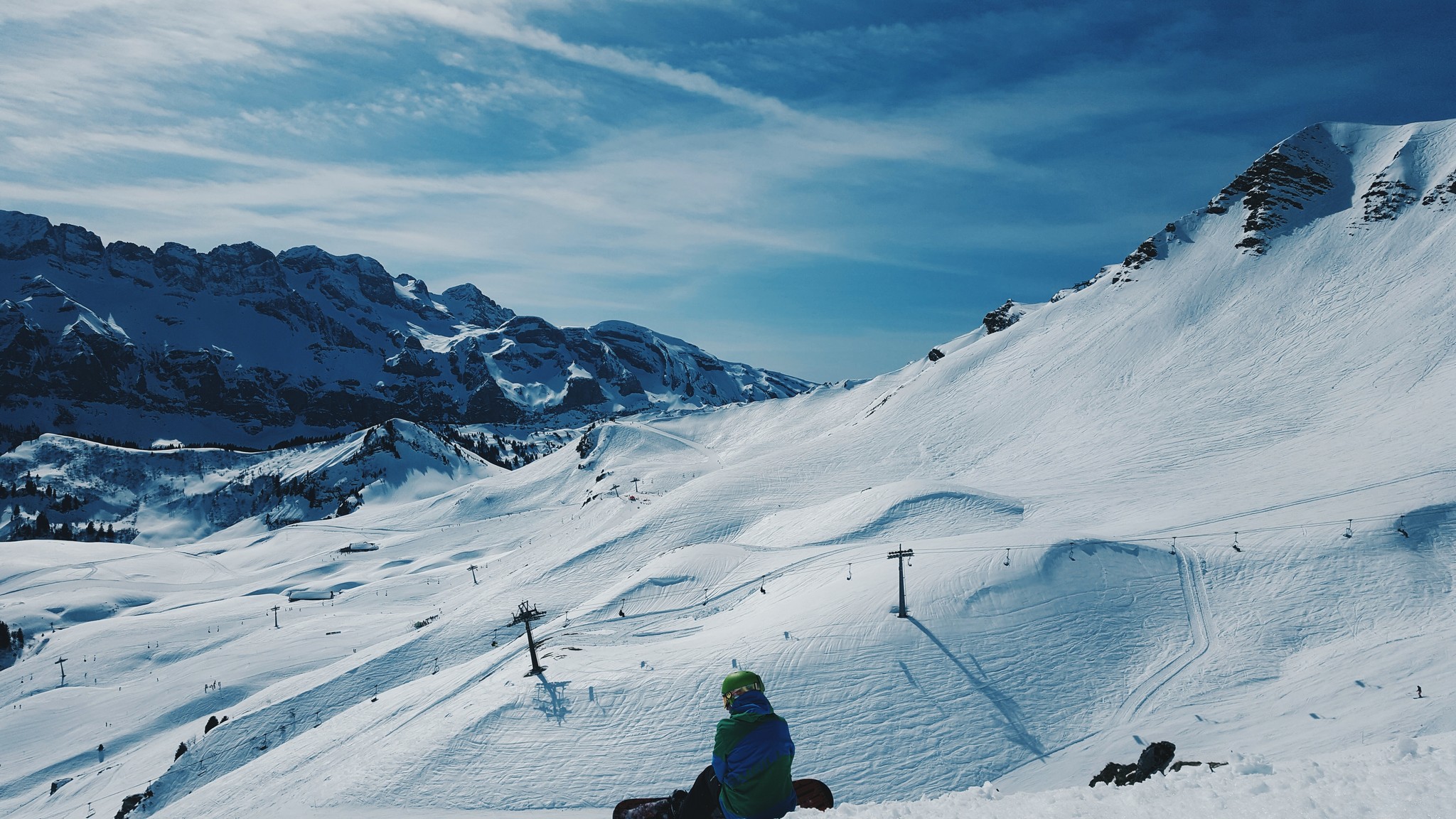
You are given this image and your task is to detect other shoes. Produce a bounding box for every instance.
[673,789,687,813]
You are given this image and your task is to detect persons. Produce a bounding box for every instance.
[712,671,797,819]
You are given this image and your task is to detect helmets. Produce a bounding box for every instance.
[721,671,764,705]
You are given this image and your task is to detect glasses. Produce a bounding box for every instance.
[722,693,729,708]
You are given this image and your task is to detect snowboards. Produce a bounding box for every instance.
[611,778,834,819]
